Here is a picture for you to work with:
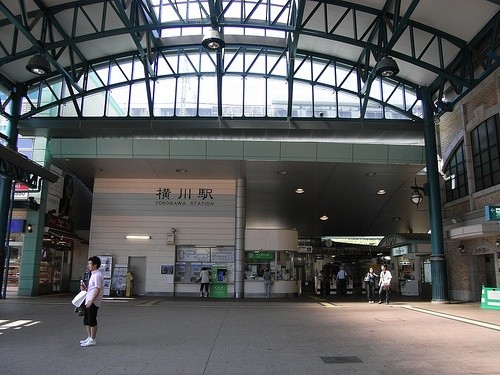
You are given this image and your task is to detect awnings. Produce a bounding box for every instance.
[50,230,89,246]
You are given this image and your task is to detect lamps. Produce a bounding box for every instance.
[495,238,500,247]
[410,177,424,206]
[458,243,464,252]
[201,27,226,50]
[375,56,399,77]
[27,222,33,233]
[25,54,52,75]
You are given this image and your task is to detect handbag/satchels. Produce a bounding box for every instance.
[74,306,86,316]
[71,290,87,308]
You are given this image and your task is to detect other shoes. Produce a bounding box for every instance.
[369,300,371,304]
[386,302,389,304]
[372,300,374,303]
[81,338,96,347]
[80,337,91,344]
[379,301,382,303]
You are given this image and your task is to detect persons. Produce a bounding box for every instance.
[79,267,91,316]
[378,264,392,304]
[283,270,290,280]
[59,174,73,216]
[320,270,327,295]
[80,256,103,346]
[200,267,209,298]
[337,267,347,297]
[365,267,378,303]
[126,271,134,297]
[263,268,271,300]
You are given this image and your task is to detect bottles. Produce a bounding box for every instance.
[81,280,87,290]
